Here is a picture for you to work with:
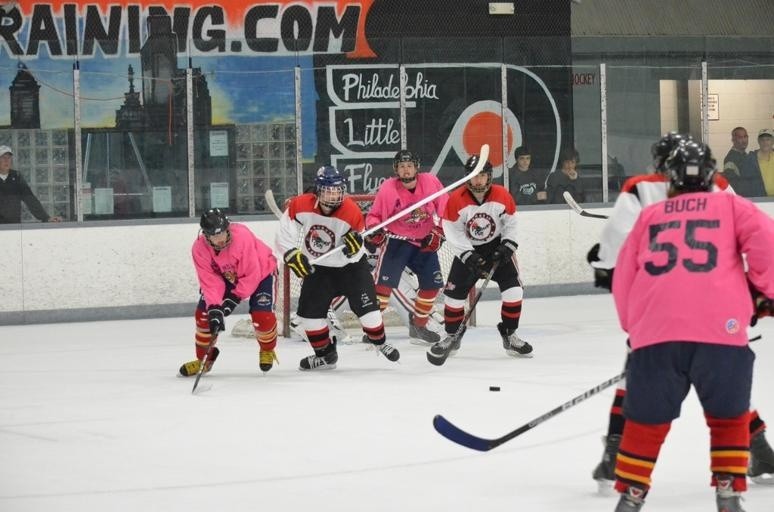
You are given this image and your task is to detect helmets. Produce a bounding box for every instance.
[464,154,493,194]
[200,207,231,250]
[391,149,421,175]
[652,132,692,173]
[665,138,717,192]
[313,165,347,209]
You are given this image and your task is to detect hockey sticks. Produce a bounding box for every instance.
[427,261,500,366]
[563,191,610,219]
[192,330,218,394]
[308,145,490,265]
[434,371,626,451]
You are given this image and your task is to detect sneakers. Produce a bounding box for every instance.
[431,326,466,354]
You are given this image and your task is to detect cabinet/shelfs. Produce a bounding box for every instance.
[236,123,296,214]
[0,129,70,222]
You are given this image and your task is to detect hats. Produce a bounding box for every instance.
[0,145,13,157]
[758,128,774,137]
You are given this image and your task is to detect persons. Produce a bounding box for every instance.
[362,148,451,345]
[500,145,548,206]
[585,129,774,481]
[430,153,534,355]
[609,137,774,511]
[180,208,278,376]
[544,146,585,204]
[276,165,400,371]
[0,144,60,225]
[723,126,749,192]
[743,128,774,197]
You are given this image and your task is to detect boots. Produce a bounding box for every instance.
[747,431,774,477]
[714,474,744,512]
[591,433,620,480]
[614,482,648,512]
[363,335,369,343]
[497,322,532,353]
[260,349,274,371]
[300,336,338,369]
[376,343,399,362]
[180,347,219,376]
[408,312,440,343]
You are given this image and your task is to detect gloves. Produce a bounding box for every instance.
[587,269,612,290]
[343,230,363,258]
[283,247,311,277]
[743,273,774,317]
[208,304,224,333]
[420,226,446,255]
[222,292,241,316]
[367,223,386,247]
[460,250,488,271]
[492,238,518,266]
